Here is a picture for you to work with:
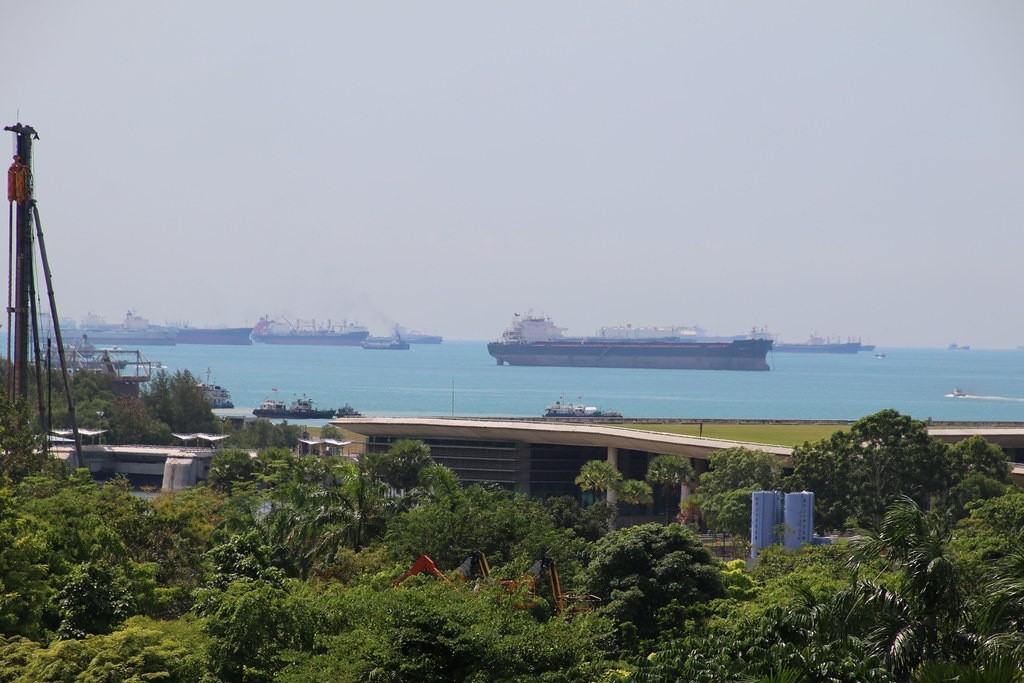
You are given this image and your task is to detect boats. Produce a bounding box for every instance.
[367,333,443,344]
[862,345,875,350]
[875,353,885,357]
[251,313,369,346]
[542,394,623,423]
[336,404,363,417]
[360,336,409,350]
[178,326,253,345]
[196,368,234,408]
[952,388,966,397]
[252,393,336,419]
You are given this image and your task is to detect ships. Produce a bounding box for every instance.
[776,335,861,354]
[487,307,775,370]
